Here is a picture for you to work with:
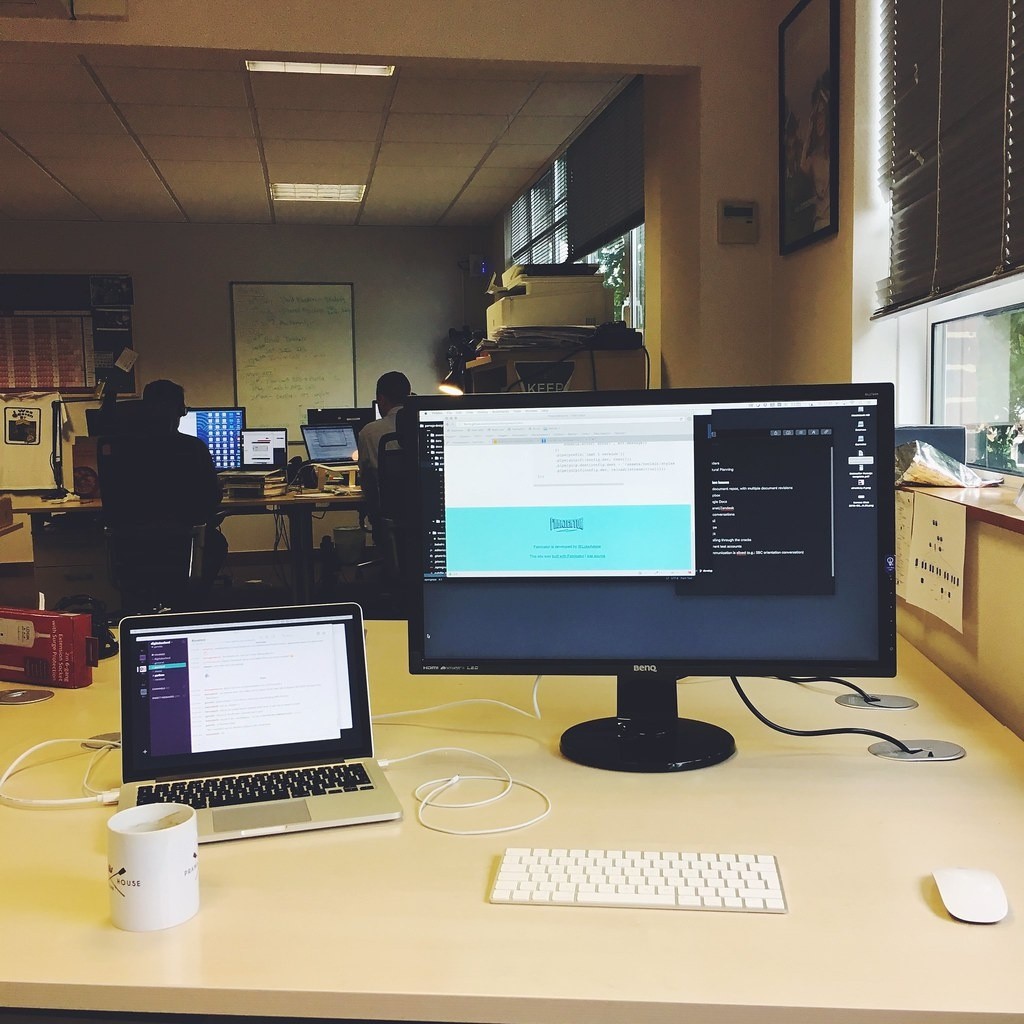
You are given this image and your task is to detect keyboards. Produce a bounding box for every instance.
[490,848,789,914]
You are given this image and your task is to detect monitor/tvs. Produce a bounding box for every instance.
[177,406,246,473]
[306,408,376,437]
[408,381,895,774]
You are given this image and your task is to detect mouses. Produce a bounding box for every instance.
[931,867,1008,924]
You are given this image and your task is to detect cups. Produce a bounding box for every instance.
[107,802,200,933]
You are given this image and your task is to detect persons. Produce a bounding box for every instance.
[358,371,410,512]
[136,379,227,611]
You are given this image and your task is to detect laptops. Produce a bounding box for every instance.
[116,602,402,843]
[217,428,288,479]
[299,423,360,472]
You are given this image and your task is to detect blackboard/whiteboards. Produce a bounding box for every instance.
[230,280,359,443]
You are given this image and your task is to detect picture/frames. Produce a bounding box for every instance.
[778,0,839,255]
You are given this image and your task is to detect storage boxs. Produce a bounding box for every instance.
[0,605,99,690]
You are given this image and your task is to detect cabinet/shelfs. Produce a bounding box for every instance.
[466,348,647,394]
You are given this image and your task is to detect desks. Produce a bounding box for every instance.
[0,618,1024,1024]
[10,483,364,612]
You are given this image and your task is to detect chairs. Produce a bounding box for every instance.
[357,432,415,595]
[97,435,233,615]
